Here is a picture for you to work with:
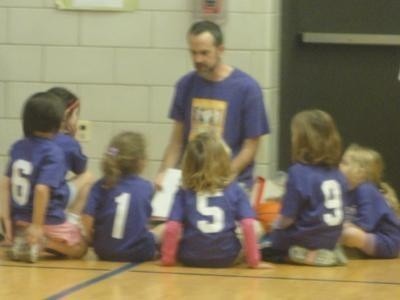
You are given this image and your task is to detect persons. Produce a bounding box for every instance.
[81,131,166,262]
[339,143,399,259]
[155,130,273,270]
[46,87,96,247]
[259,109,350,267]
[155,20,272,201]
[0,92,87,264]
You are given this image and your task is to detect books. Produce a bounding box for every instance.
[148,169,183,220]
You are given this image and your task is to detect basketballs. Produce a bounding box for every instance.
[257,202,281,229]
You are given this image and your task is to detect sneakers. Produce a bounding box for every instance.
[7,235,40,264]
[287,244,348,268]
